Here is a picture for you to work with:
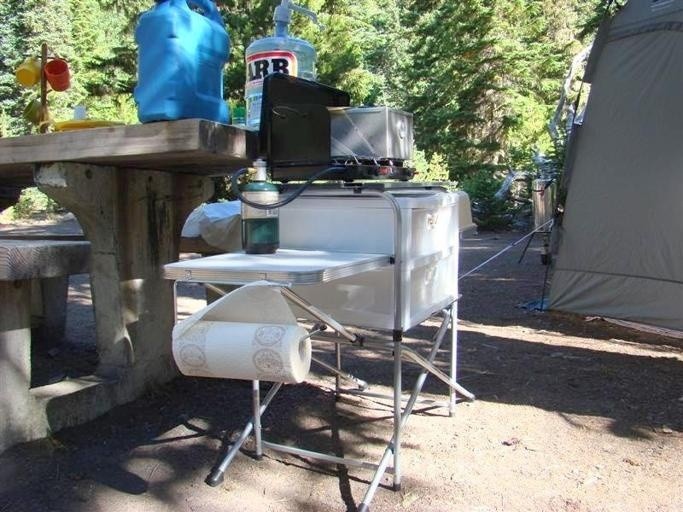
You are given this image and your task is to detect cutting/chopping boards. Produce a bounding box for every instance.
[55,120,124,131]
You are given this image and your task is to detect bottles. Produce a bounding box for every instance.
[540,231,551,264]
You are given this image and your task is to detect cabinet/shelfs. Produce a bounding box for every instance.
[208,187,475,512]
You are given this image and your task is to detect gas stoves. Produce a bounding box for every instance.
[271,160,416,183]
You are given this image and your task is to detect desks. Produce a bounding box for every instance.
[0,116,258,410]
[162,245,402,491]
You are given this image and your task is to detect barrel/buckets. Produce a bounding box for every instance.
[133,0,232,126]
[539,237,552,265]
[245,0,318,133]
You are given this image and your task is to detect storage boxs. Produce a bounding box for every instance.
[328,107,415,166]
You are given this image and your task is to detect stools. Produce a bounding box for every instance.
[0,237,90,447]
